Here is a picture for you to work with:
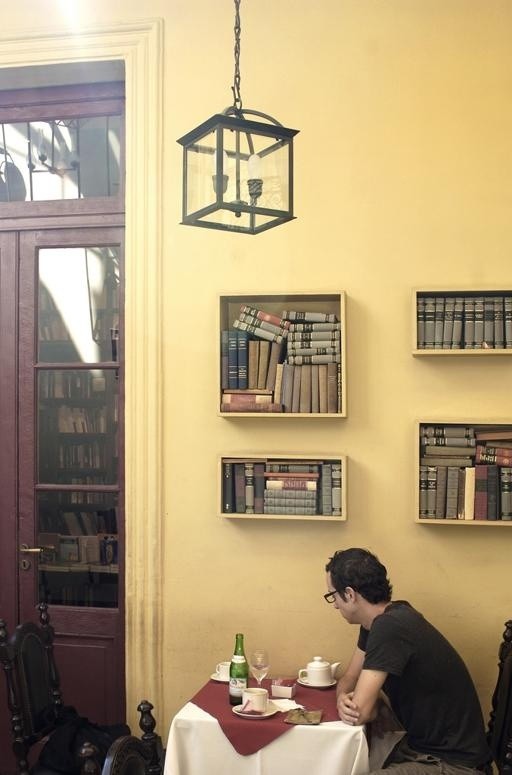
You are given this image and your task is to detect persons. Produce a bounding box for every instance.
[323,547,485,775]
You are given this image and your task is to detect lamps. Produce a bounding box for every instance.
[28,120,80,175]
[175,0,300,235]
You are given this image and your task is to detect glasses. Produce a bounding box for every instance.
[324,584,356,603]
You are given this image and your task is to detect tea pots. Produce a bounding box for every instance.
[299,656,341,684]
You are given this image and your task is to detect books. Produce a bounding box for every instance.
[416,296,512,349]
[34,246,123,575]
[221,304,342,413]
[419,425,512,522]
[222,458,342,517]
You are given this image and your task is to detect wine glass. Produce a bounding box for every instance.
[249,648,271,687]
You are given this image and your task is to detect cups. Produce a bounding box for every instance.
[242,688,268,712]
[216,662,231,679]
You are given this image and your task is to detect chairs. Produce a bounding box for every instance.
[0,602,131,775]
[102,700,165,775]
[484,619,512,775]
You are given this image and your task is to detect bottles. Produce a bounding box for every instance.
[229,634,249,706]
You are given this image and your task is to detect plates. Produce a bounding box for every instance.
[210,673,229,682]
[232,704,277,719]
[296,679,337,688]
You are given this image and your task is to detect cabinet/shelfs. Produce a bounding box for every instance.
[216,289,347,417]
[410,285,512,355]
[0,198,124,727]
[217,454,348,521]
[412,416,512,525]
